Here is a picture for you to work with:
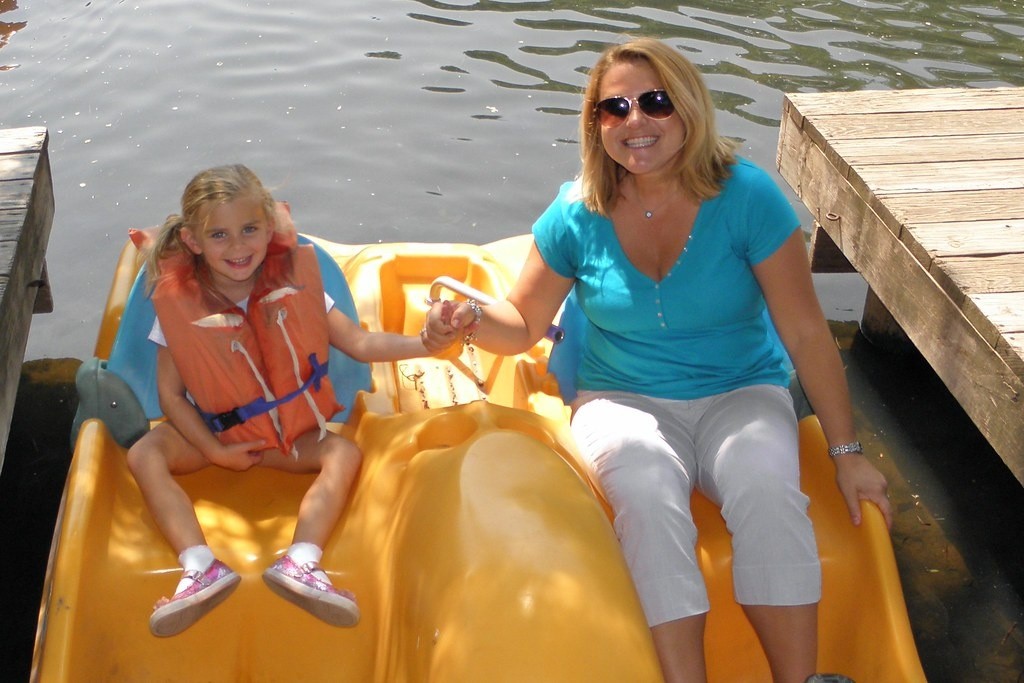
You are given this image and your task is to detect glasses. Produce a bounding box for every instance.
[594,89,676,129]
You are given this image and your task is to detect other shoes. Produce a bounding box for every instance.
[261,555,359,628]
[149,558,242,638]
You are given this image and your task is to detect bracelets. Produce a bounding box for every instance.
[465,299,483,352]
[827,442,864,458]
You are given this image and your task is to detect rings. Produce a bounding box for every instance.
[420,328,428,336]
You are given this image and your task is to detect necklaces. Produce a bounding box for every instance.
[634,181,679,218]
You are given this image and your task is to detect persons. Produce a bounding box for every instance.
[427,35,894,683]
[124,164,458,638]
